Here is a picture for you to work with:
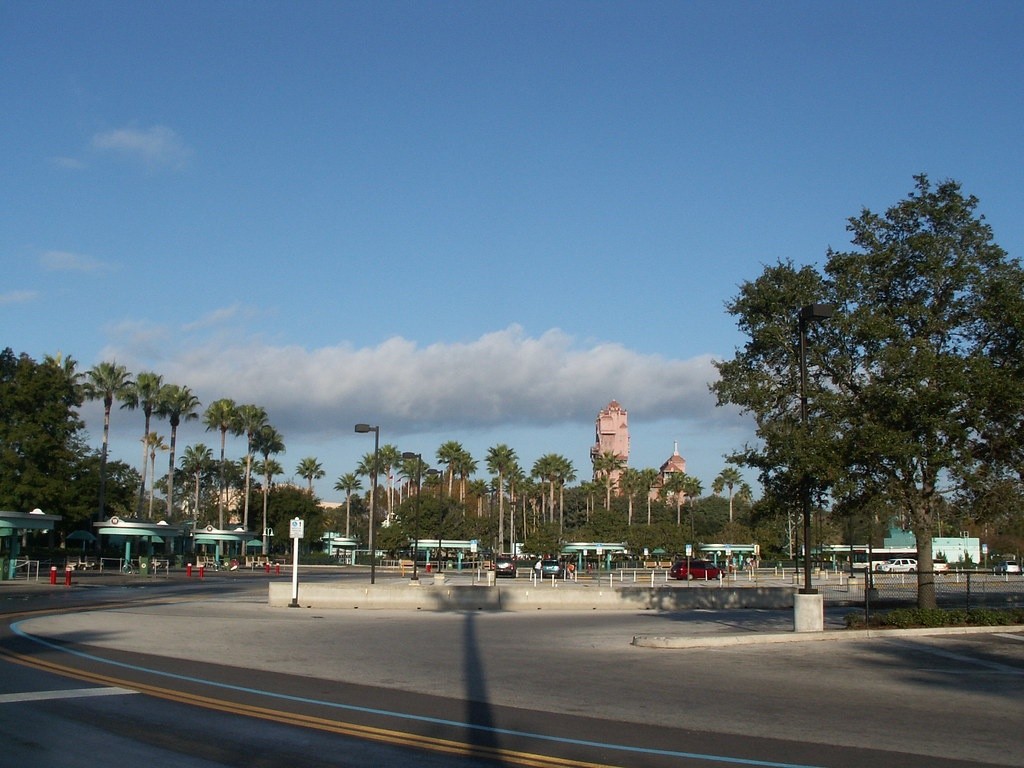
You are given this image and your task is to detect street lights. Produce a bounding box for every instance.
[263,526,274,562]
[487,489,497,571]
[402,452,421,580]
[510,501,517,557]
[354,423,379,585]
[793,303,837,634]
[425,468,443,573]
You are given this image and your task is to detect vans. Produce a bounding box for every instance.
[876,558,918,574]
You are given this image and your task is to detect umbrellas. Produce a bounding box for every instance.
[140,535,165,556]
[196,539,217,555]
[67,531,97,557]
[247,539,263,555]
[651,548,666,568]
[561,549,578,553]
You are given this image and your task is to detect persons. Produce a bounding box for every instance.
[567,562,574,579]
[533,559,542,578]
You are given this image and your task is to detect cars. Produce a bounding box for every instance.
[671,558,725,581]
[534,559,563,579]
[992,561,1021,575]
[931,559,949,576]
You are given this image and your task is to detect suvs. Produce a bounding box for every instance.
[495,557,517,578]
